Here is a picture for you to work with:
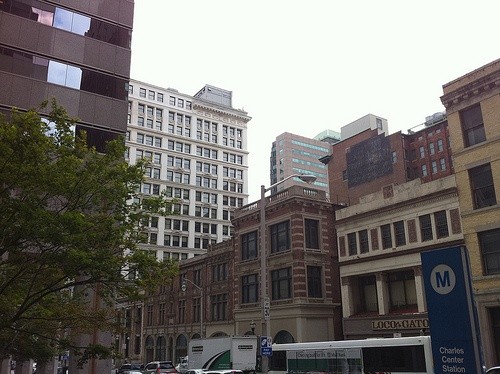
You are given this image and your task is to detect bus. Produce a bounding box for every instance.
[268,333,435,374]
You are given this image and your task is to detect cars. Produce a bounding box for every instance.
[10,360,64,374]
[111,356,244,374]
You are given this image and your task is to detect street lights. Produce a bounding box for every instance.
[250,319,256,335]
[261,172,317,325]
[184,278,203,341]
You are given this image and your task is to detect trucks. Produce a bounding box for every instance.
[176,334,257,373]
